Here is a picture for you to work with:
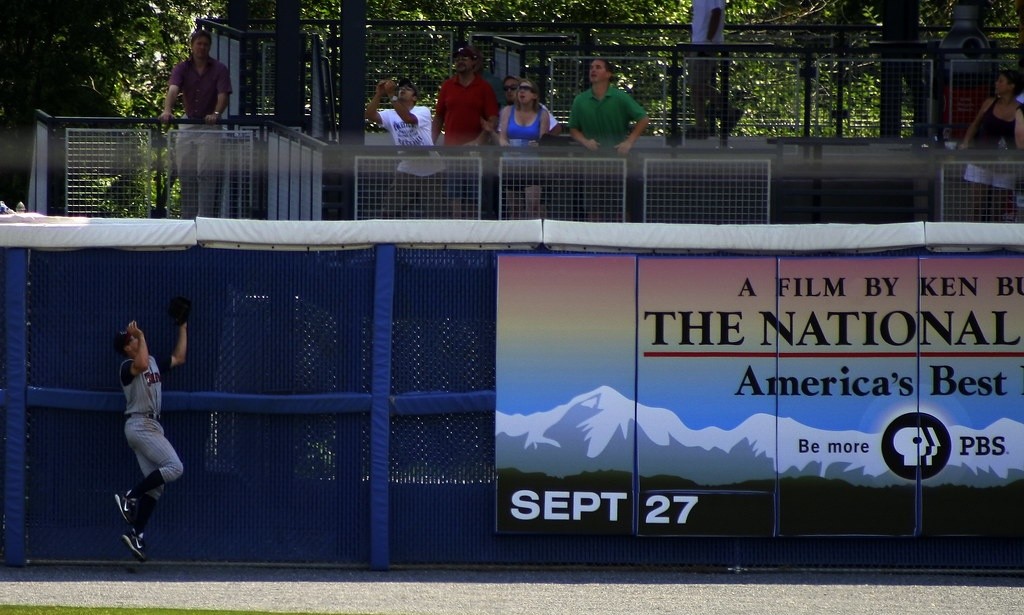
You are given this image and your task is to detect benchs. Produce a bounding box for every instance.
[153,134,1023,221]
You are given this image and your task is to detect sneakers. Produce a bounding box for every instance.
[116,490,136,524]
[122,530,145,559]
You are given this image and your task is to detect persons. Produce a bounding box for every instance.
[432,46,500,220]
[567,58,649,222]
[159,31,233,220]
[684,0,743,141]
[365,76,450,219]
[480,76,563,221]
[114,295,192,559]
[959,70,1024,223]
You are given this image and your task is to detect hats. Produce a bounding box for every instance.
[399,78,419,97]
[453,48,473,57]
[114,331,132,351]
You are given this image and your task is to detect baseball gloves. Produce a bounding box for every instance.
[167,296,193,324]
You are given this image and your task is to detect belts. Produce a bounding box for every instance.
[126,413,160,421]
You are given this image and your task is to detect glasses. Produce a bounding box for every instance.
[519,85,532,91]
[503,84,517,91]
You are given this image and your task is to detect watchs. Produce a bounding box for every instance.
[390,96,398,103]
[214,112,219,115]
